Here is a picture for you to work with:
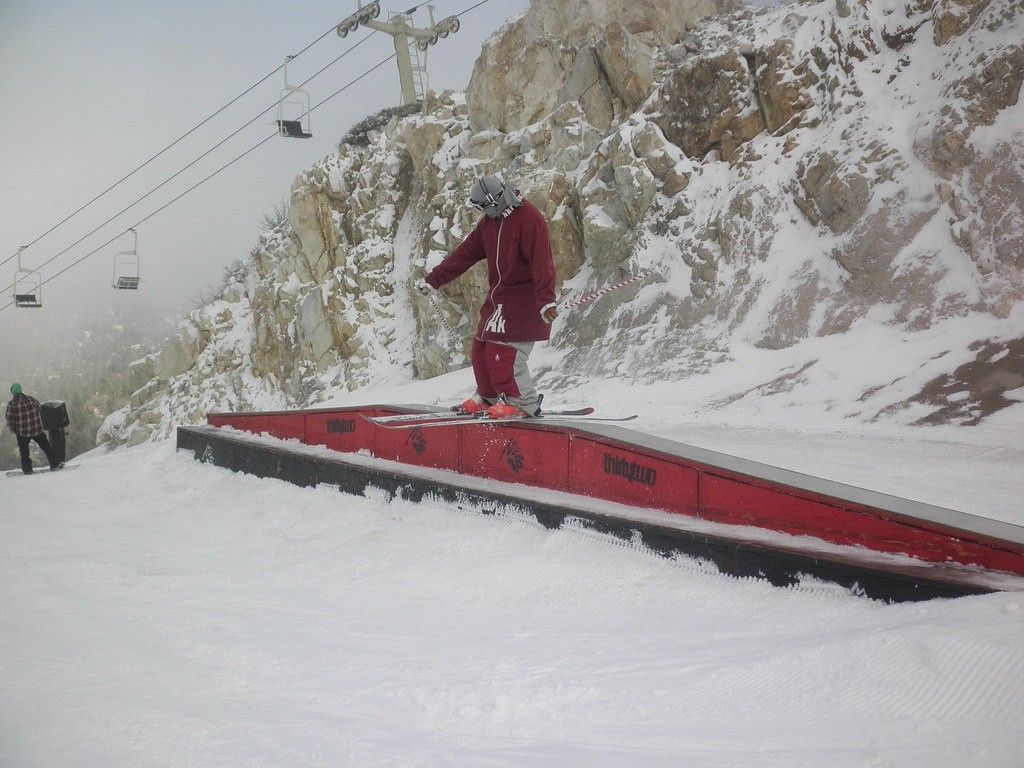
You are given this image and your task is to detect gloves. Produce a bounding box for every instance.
[418,282,430,296]
[542,307,557,323]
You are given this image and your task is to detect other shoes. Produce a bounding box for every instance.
[51,462,64,471]
[23,469,33,475]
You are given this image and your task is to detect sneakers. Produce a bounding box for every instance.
[486,400,529,419]
[455,394,493,416]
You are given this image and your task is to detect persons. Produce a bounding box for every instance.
[415,174,559,419]
[6,383,64,474]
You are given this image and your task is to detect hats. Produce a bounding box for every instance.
[10,384,22,395]
[471,175,520,218]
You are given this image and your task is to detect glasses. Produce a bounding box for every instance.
[469,198,484,213]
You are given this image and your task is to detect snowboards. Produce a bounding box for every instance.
[5,463,82,478]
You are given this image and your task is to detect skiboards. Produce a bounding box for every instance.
[357,406,640,430]
